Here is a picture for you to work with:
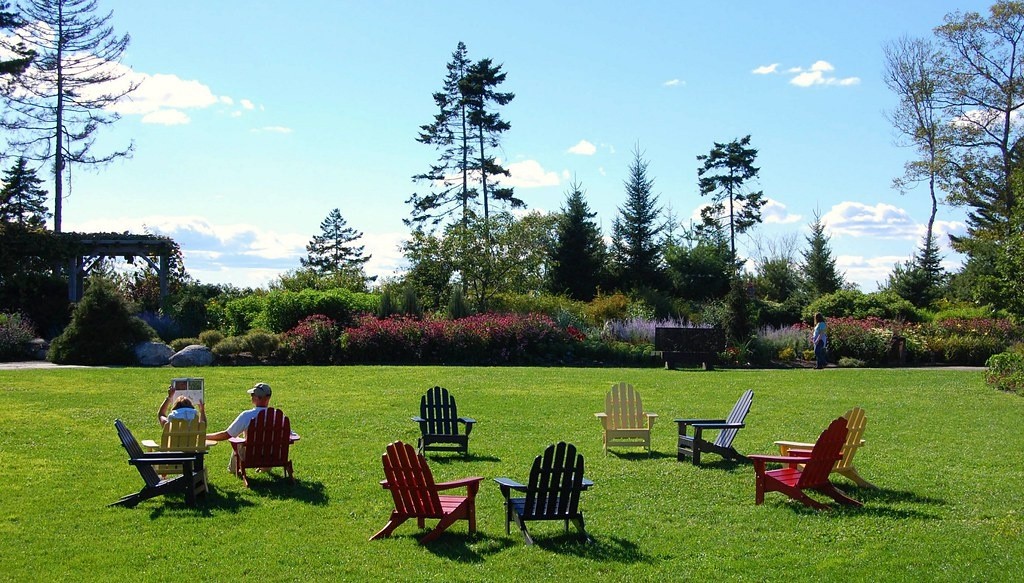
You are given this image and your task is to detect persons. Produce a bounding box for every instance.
[812,313,827,369]
[157,386,206,477]
[206,383,285,473]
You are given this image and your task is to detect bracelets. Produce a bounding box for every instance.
[166,396,172,401]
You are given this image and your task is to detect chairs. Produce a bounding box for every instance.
[142,418,219,494]
[109,418,210,510]
[595,382,658,458]
[493,441,596,548]
[672,389,754,466]
[747,416,862,513]
[228,407,300,488]
[774,407,878,499]
[412,386,476,460]
[368,441,485,546]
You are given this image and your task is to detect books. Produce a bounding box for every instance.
[171,377,204,405]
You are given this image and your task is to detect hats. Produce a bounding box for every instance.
[247,383,271,397]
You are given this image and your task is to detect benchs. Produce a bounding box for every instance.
[654,327,726,370]
